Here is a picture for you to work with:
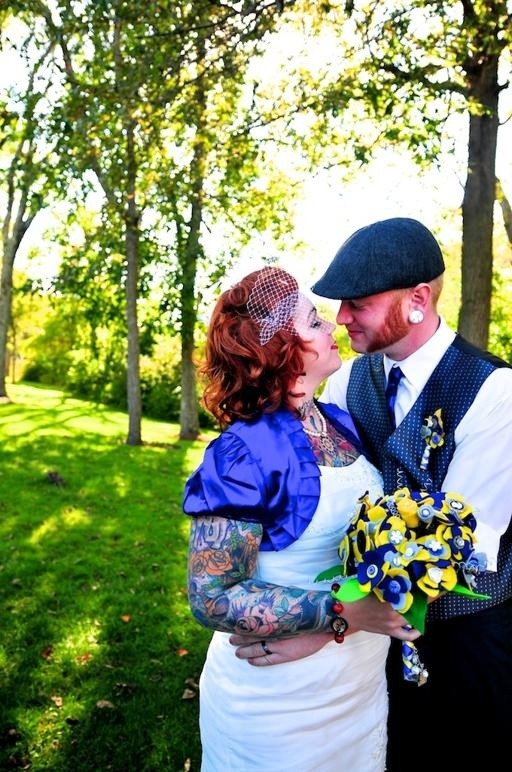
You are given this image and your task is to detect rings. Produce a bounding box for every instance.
[260,640,272,655]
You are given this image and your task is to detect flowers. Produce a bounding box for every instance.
[418,408,448,472]
[312,486,493,688]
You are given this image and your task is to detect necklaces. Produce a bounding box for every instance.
[302,400,337,456]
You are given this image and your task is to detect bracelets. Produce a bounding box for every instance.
[330,582,348,643]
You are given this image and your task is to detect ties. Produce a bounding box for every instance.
[382,365,404,419]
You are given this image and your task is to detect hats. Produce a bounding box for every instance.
[310,218,446,300]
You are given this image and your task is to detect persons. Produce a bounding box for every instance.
[230,217,512,772]
[183,265,420,772]
